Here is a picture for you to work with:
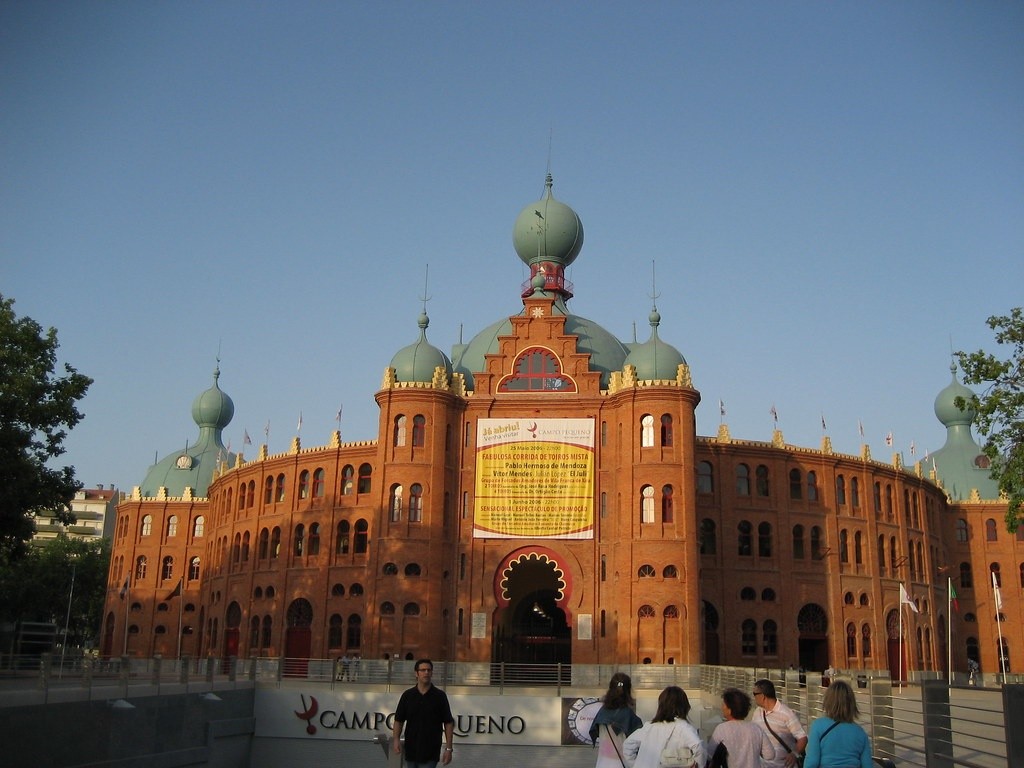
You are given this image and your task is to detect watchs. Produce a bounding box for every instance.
[791,751,802,760]
[446,747,453,752]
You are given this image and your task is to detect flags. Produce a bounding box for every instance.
[163,580,181,601]
[899,583,918,613]
[118,578,129,599]
[216,403,343,462]
[949,579,958,611]
[718,398,939,473]
[992,571,1003,609]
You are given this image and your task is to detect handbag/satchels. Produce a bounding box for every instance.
[658,746,697,768]
[795,749,806,768]
[710,741,730,768]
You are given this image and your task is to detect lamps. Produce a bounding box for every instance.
[533,602,550,619]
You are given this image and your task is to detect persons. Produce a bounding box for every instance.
[392,659,455,768]
[588,672,643,768]
[803,680,874,768]
[623,686,708,768]
[971,668,978,686]
[704,687,776,768]
[336,651,351,682]
[827,664,835,684]
[750,679,808,768]
[350,652,363,681]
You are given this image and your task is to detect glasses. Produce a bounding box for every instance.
[418,668,432,673]
[752,691,764,696]
